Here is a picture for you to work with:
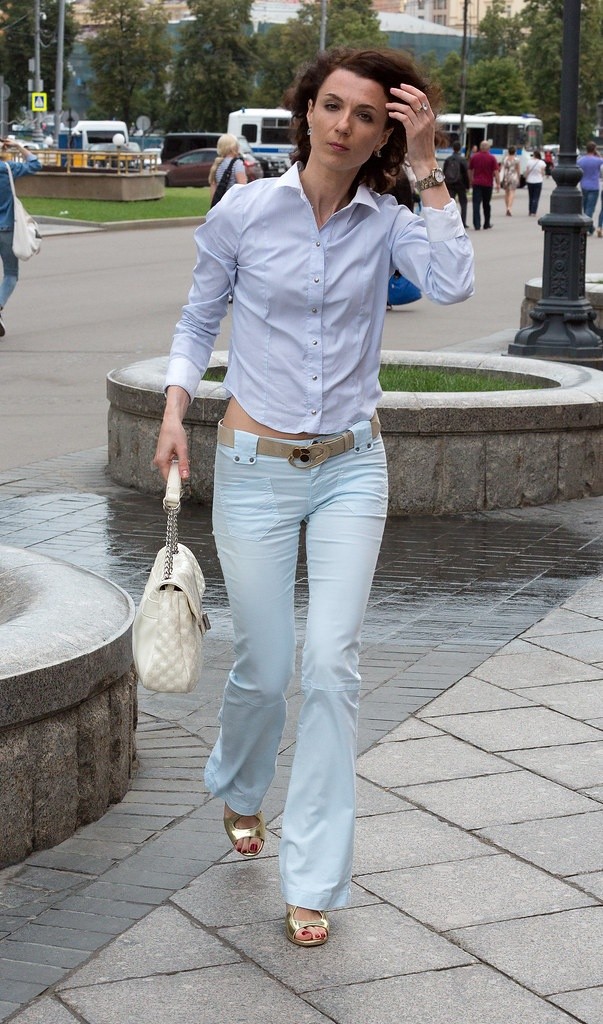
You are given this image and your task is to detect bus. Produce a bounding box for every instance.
[227,108,543,189]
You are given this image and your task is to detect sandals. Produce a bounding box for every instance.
[285,904,329,946]
[223,813,265,855]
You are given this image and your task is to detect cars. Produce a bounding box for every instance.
[251,154,287,178]
[154,148,218,187]
[542,144,580,168]
[5,135,44,171]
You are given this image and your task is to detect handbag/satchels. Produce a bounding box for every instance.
[132,461,211,692]
[12,198,42,261]
[210,185,225,210]
[518,176,525,189]
[388,270,421,306]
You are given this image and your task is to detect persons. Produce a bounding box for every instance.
[525,151,547,216]
[545,150,554,175]
[576,141,603,238]
[208,134,248,304]
[442,140,500,230]
[0,137,43,336]
[502,146,521,216]
[155,46,476,950]
[471,145,477,158]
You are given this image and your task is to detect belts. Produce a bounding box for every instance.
[217,410,381,470]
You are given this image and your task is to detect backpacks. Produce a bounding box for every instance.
[444,156,464,188]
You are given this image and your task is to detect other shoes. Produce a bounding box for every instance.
[506,210,511,217]
[463,225,469,228]
[596,227,602,237]
[488,224,493,228]
[0,322,5,337]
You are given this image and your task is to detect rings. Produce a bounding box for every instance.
[416,103,428,113]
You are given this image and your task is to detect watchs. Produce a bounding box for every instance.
[415,168,446,192]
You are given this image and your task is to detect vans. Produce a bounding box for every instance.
[43,120,129,152]
[159,133,227,164]
[127,136,165,169]
